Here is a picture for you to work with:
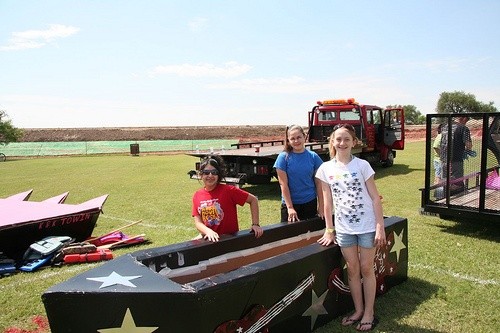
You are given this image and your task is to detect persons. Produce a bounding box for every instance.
[433,117,473,194]
[314,123,388,331]
[191,155,264,242]
[393,117,397,123]
[273,124,325,224]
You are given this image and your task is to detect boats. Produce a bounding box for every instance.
[41,215,408,333]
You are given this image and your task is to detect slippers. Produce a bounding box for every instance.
[340,314,379,331]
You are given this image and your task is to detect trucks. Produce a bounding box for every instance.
[185,99,404,189]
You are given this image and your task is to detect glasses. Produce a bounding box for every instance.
[202,170,218,175]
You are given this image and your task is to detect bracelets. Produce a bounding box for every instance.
[324,229,336,235]
[251,223,260,227]
